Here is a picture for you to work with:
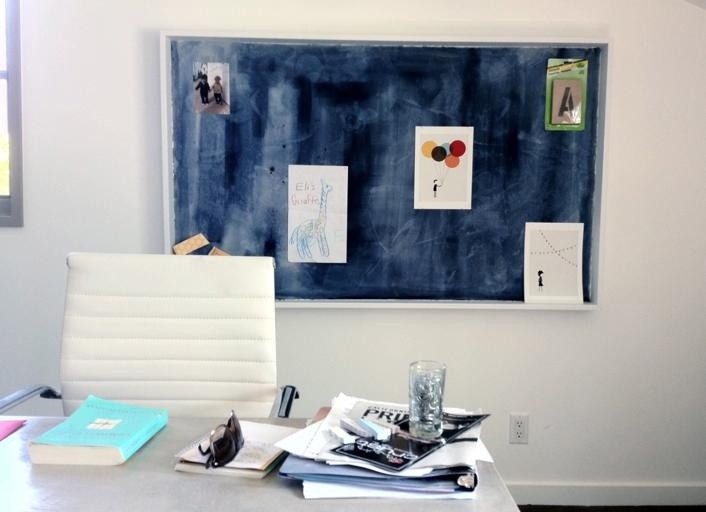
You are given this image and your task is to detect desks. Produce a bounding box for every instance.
[0,416,522,512]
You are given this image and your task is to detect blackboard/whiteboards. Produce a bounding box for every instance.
[159,34,608,312]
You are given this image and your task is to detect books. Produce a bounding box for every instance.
[173,420,305,478]
[28,394,169,466]
[298,479,477,501]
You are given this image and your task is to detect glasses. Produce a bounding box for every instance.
[198,413,245,470]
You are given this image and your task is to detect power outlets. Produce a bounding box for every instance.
[509,413,528,443]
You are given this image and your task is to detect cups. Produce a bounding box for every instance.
[408,358,449,440]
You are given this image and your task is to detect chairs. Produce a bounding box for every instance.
[1,252,300,416]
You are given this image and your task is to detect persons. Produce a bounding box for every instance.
[195,74,210,105]
[209,75,223,106]
